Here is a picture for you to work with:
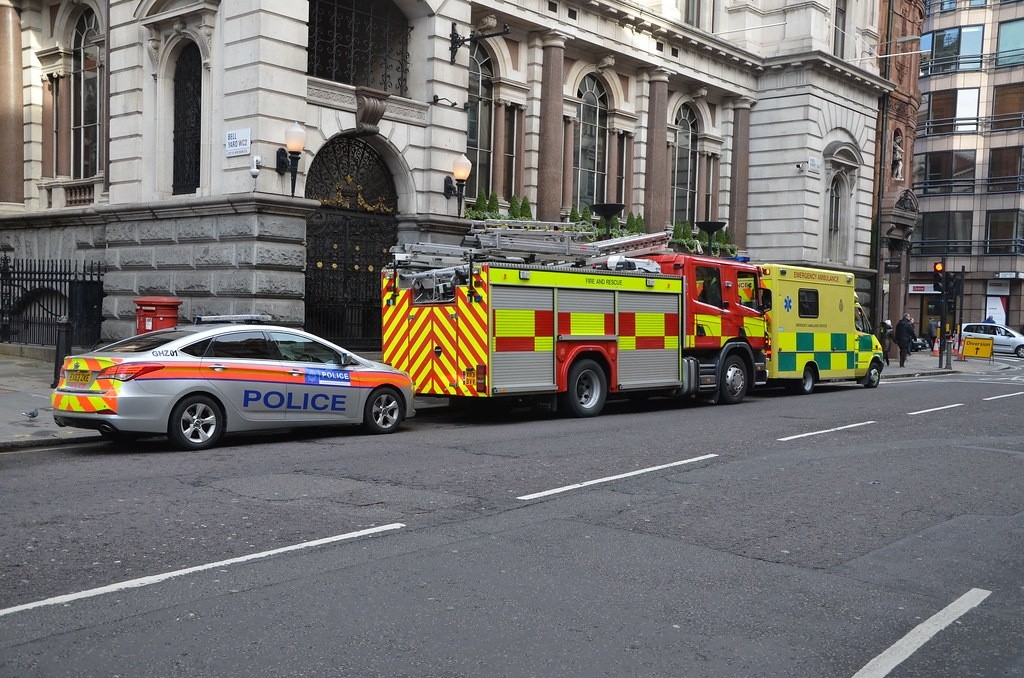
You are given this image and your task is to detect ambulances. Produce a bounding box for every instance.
[696,261,885,395]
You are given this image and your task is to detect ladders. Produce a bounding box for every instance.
[387,217,673,270]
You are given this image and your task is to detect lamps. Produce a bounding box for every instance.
[443,154,472,218]
[277,121,306,197]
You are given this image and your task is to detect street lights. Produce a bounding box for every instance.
[694,220,727,256]
[590,203,627,239]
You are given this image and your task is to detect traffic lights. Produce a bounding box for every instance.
[933,262,944,291]
[946,271,956,293]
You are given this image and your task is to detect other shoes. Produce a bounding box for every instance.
[899,362,904,367]
[887,360,889,366]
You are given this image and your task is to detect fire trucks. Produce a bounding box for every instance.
[378,220,775,417]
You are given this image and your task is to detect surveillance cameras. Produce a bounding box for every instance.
[250,169,259,178]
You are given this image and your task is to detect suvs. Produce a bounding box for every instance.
[952,321,1024,358]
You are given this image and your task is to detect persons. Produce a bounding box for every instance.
[980,315,996,334]
[894,312,917,367]
[892,135,905,179]
[908,317,915,355]
[878,319,898,366]
[926,317,938,351]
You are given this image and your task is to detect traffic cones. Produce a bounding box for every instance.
[950,334,968,362]
[929,338,939,357]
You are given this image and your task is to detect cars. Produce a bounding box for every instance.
[910,333,929,352]
[50,311,418,450]
